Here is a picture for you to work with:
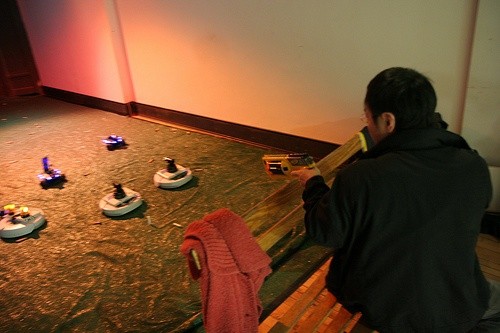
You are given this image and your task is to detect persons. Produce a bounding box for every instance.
[290,67,500,333]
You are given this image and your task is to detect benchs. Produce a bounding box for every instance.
[191,127,382,333]
